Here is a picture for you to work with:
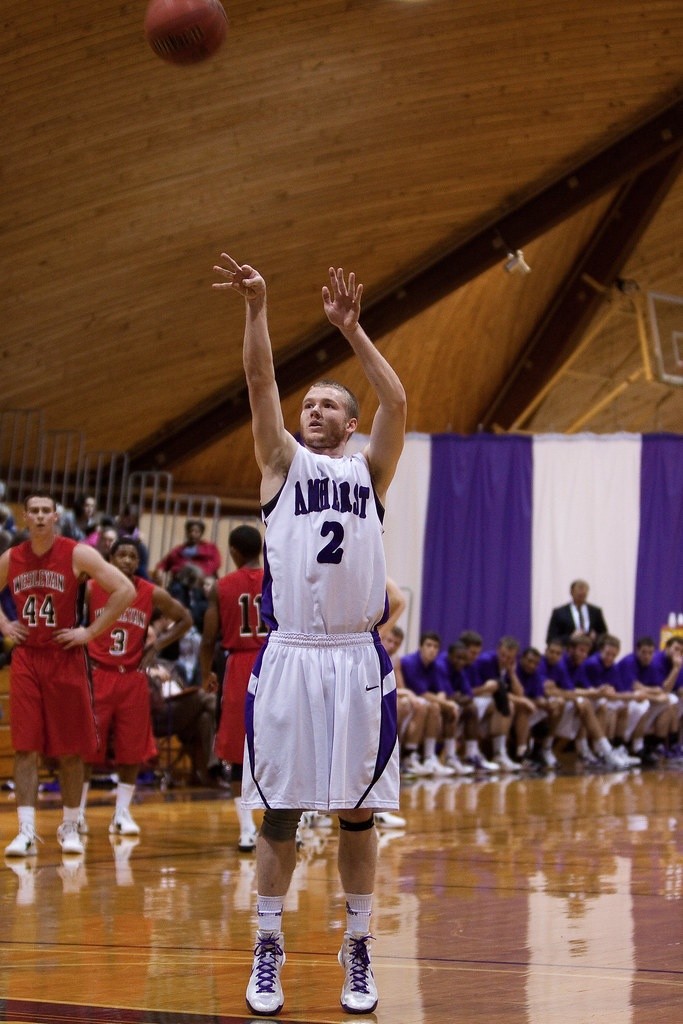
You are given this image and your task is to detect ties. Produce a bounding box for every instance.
[578,607,584,630]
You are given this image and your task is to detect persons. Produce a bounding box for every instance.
[211,252,409,1015]
[0,481,683,854]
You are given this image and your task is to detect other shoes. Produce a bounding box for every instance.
[187,771,231,791]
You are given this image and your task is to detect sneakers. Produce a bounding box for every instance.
[237,825,258,850]
[77,814,88,835]
[107,813,139,837]
[293,812,333,852]
[402,743,683,775]
[4,825,44,856]
[57,824,82,855]
[243,931,286,1015]
[337,931,378,1013]
[373,812,407,830]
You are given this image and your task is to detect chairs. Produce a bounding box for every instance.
[147,685,205,796]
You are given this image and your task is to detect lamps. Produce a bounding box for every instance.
[503,249,531,275]
